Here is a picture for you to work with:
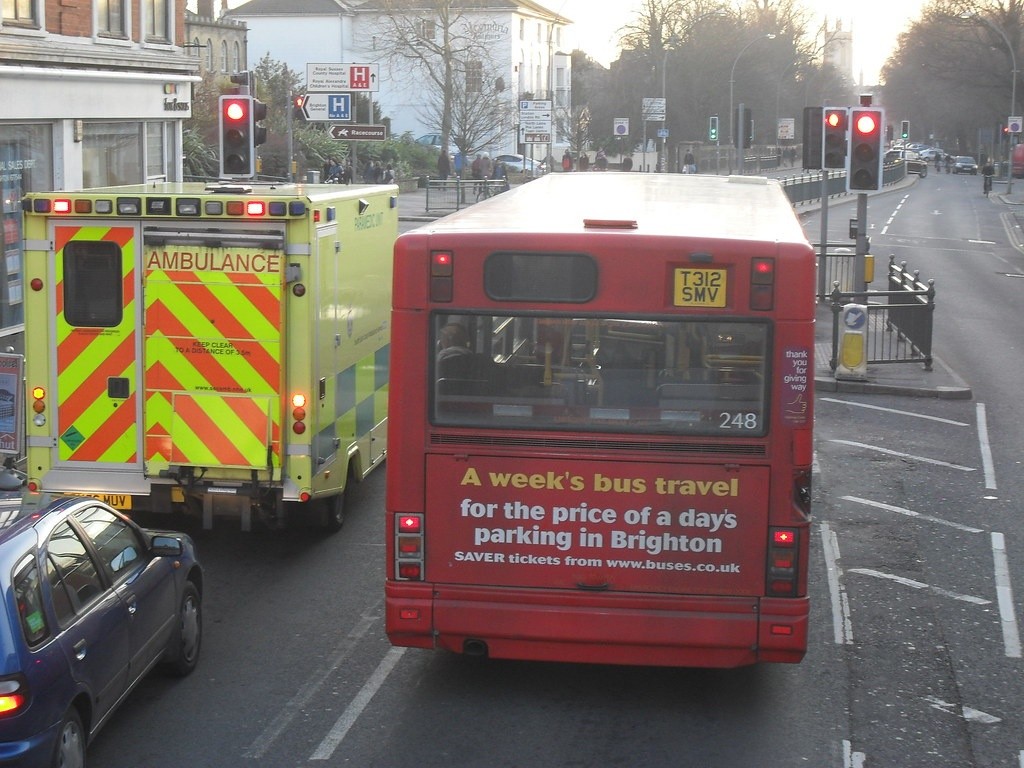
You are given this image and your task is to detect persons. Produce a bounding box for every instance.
[436,322,475,375]
[454,150,468,179]
[471,153,509,196]
[540,152,554,172]
[437,150,451,191]
[933,152,953,168]
[321,158,354,185]
[623,154,633,172]
[562,149,573,172]
[980,161,995,193]
[600,155,608,171]
[580,152,589,172]
[363,160,394,184]
[684,149,694,174]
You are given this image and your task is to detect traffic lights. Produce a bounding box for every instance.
[709,117,718,141]
[901,121,910,140]
[295,98,303,108]
[218,95,255,180]
[821,107,849,172]
[253,98,267,148]
[846,107,885,194]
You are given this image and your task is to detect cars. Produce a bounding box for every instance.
[493,155,551,184]
[415,135,473,162]
[892,142,946,162]
[883,150,928,178]
[952,155,978,175]
[0,491,204,768]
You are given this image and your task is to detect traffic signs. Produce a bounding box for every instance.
[519,100,551,144]
[326,123,386,142]
[300,93,352,121]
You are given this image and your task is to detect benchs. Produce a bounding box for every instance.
[655,367,764,411]
[436,355,602,416]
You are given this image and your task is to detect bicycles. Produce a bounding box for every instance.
[982,177,995,198]
[476,184,502,203]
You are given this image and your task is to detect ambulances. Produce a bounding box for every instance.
[17,179,399,532]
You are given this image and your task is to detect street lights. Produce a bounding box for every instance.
[729,34,776,176]
[959,13,1016,194]
[663,11,727,172]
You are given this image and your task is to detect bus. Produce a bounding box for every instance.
[385,170,817,666]
